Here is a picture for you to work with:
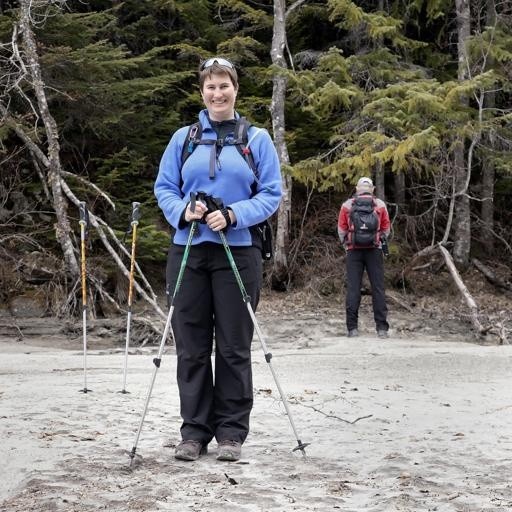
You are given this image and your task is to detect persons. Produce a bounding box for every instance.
[153,58,283,460]
[336,176,393,340]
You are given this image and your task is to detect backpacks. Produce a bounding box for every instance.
[340,194,389,256]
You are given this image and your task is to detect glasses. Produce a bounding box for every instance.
[202,57,233,69]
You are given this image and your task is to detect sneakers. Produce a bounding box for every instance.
[216,439,241,461]
[348,329,359,337]
[174,439,207,460]
[377,330,390,339]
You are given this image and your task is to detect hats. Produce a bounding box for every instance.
[356,177,373,186]
